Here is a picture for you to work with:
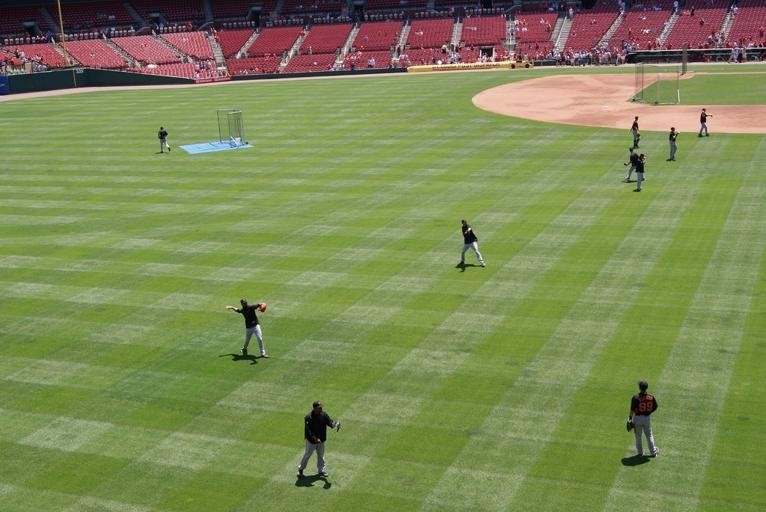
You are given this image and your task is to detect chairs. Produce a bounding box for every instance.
[0,2,766,78]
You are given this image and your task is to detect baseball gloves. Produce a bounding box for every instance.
[258,303,266,313]
[626,420,634,432]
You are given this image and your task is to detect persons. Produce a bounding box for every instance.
[635,154,644,191]
[627,380,659,457]
[629,116,640,148]
[226,298,269,358]
[698,108,712,137]
[158,127,171,153]
[456,220,486,267]
[2,1,766,80]
[669,127,680,161]
[624,148,639,179]
[296,400,342,477]
